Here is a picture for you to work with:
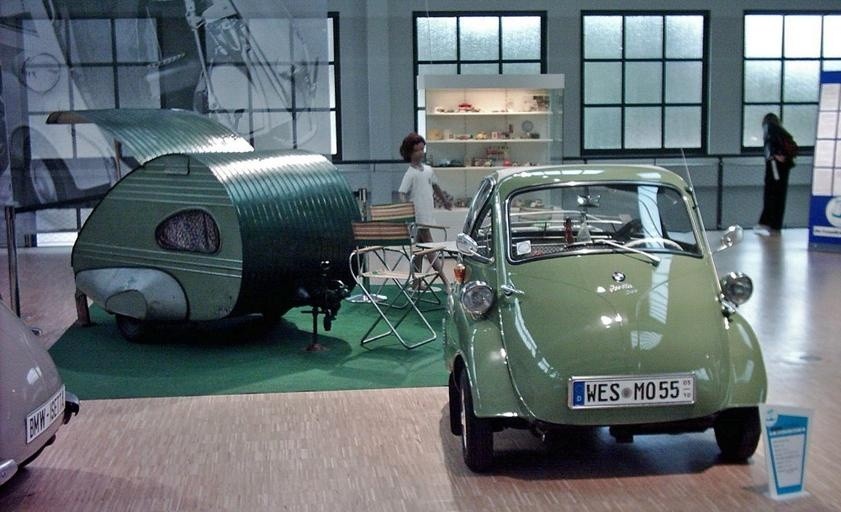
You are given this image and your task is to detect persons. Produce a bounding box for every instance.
[752,112,800,237]
[397,132,455,297]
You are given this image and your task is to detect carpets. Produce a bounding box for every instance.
[47,283,450,401]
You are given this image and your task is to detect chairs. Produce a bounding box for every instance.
[348,200,554,350]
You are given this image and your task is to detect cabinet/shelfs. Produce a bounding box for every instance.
[416,73,566,258]
